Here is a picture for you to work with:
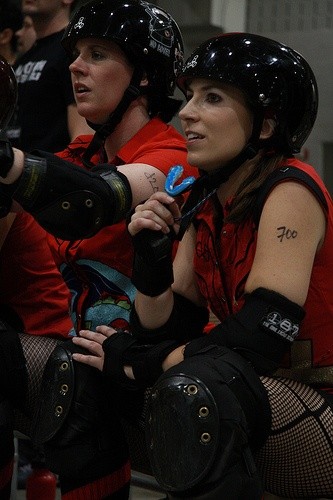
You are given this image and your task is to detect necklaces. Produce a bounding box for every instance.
[175,188,216,222]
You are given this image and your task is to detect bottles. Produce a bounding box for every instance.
[27,463,56,500]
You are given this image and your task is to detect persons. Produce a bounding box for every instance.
[36,33,333,500]
[0,0,200,500]
[0,0,96,154]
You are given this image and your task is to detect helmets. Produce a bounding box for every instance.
[59,0,184,98]
[175,32,319,156]
[0,55,18,129]
